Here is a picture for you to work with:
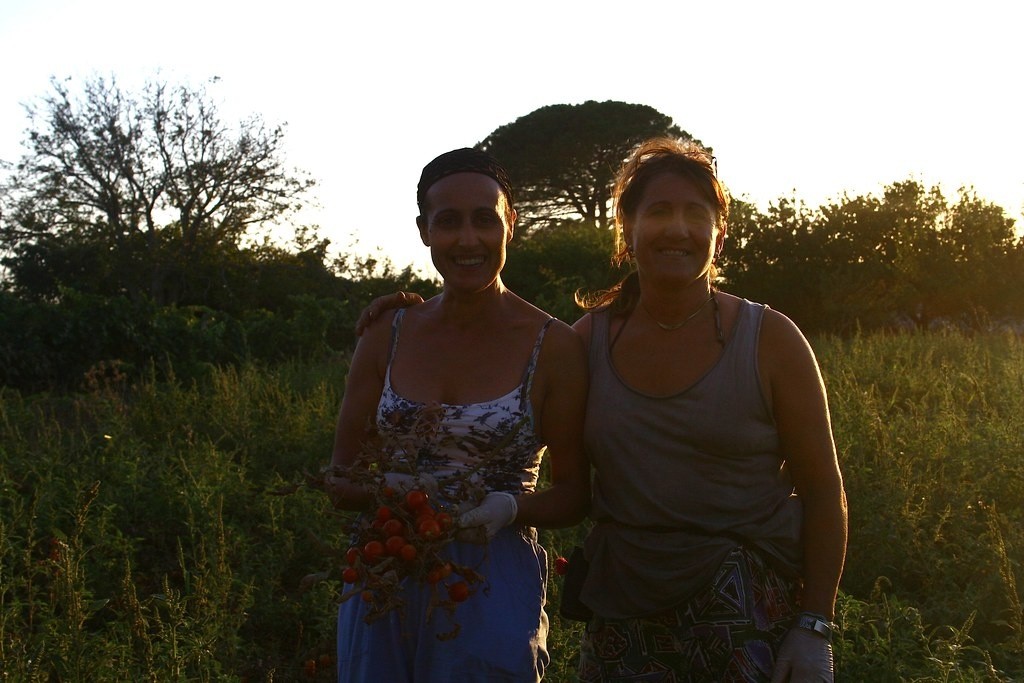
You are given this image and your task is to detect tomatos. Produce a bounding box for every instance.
[342,488,569,601]
[304,653,329,670]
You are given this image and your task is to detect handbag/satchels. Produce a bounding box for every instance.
[560,546,594,624]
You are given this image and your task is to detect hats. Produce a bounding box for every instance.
[417,147,515,218]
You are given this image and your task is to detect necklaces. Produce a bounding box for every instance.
[639,294,714,331]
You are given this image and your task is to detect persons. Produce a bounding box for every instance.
[355,137,849,683]
[329,148,588,683]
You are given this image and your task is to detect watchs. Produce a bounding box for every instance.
[792,614,832,639]
